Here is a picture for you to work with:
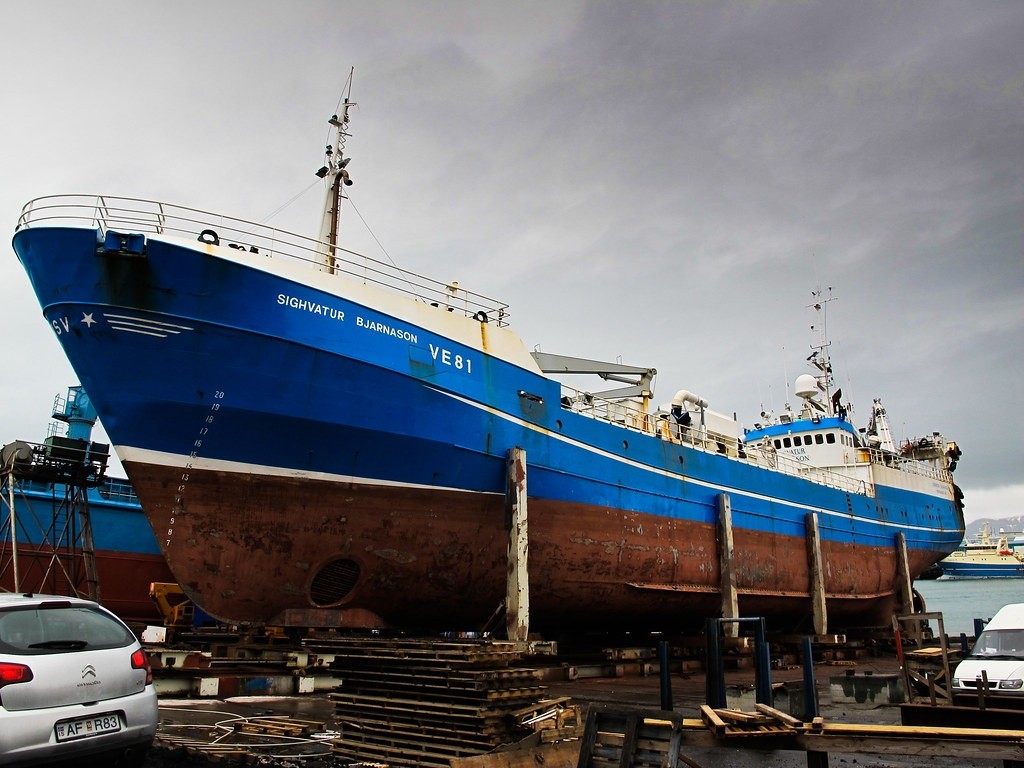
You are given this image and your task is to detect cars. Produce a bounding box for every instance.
[0,592,158,768]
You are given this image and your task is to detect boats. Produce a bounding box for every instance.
[1,386,218,641]
[937,538,1024,579]
[13,65,966,644]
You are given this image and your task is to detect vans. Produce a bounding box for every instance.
[950,603,1024,710]
